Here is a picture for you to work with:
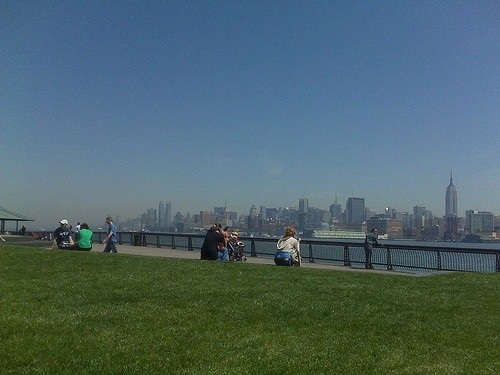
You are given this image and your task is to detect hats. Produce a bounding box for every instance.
[59,219,68,224]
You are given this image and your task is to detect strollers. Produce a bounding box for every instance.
[227,241,247,262]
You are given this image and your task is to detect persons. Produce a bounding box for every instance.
[364,228,380,269]
[200,224,229,260]
[46,219,94,251]
[21,225,26,235]
[103,217,118,253]
[274,226,300,266]
